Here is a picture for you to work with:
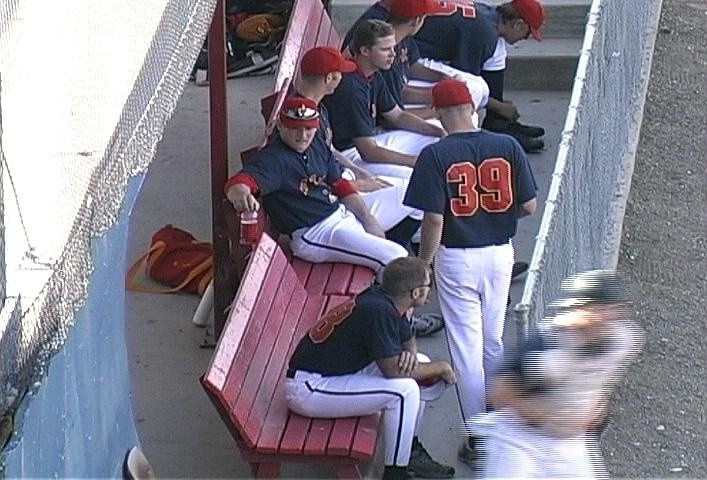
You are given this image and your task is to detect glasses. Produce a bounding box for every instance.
[421,280,433,289]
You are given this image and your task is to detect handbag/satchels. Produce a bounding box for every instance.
[125,223,214,296]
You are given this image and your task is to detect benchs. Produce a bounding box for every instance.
[197,231,392,480]
[241,0,394,298]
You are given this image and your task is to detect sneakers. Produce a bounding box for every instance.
[412,313,444,337]
[511,261,528,278]
[457,437,477,470]
[407,436,455,478]
[514,133,544,151]
[513,122,545,137]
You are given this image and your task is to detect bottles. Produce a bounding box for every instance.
[238,211,258,247]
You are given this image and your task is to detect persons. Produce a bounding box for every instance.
[461,268,643,480]
[341,1,491,131]
[286,46,435,272]
[320,18,448,179]
[402,79,539,471]
[341,1,546,154]
[505,261,528,307]
[283,256,460,480]
[222,97,445,339]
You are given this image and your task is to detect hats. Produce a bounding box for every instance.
[430,78,472,110]
[278,97,321,130]
[510,0,544,42]
[419,378,450,402]
[549,268,632,308]
[301,47,357,76]
[390,1,440,18]
